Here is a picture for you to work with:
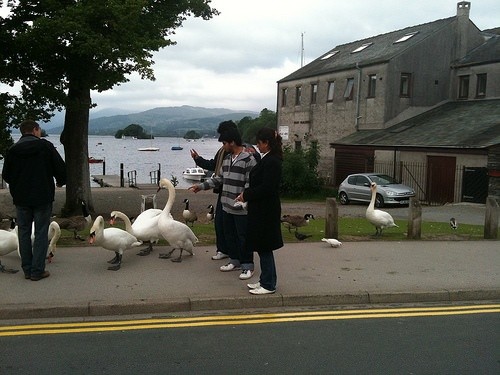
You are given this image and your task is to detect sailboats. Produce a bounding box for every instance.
[121,134,126,140]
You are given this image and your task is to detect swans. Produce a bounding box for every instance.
[157,178,199,262]
[0,225,22,273]
[366,182,400,237]
[31,220,61,264]
[89,216,141,271]
[110,208,174,256]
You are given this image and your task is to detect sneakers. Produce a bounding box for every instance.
[239,269,254,280]
[246,282,261,288]
[211,251,228,260]
[249,287,276,295]
[220,263,243,271]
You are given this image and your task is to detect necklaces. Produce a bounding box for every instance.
[230,152,242,167]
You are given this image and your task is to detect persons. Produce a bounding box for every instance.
[191,121,260,259]
[187,128,258,280]
[234,127,284,294]
[2,121,66,281]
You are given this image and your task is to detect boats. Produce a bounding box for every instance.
[181,167,206,181]
[172,146,183,150]
[138,148,160,151]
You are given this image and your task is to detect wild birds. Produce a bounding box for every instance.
[206,204,217,224]
[450,217,458,230]
[321,237,343,249]
[50,200,92,241]
[182,199,197,227]
[294,231,312,240]
[280,214,315,233]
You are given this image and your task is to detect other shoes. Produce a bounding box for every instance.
[31,271,49,281]
[25,272,31,278]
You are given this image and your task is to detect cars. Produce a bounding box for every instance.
[337,173,415,207]
